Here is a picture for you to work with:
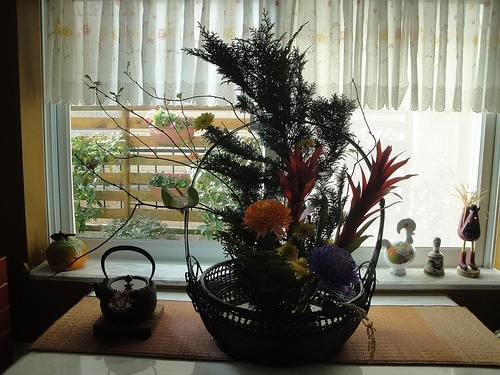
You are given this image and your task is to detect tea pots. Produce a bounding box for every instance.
[92,245,157,319]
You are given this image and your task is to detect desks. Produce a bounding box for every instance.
[2,293,500,375]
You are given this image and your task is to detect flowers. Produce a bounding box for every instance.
[32,9,419,314]
